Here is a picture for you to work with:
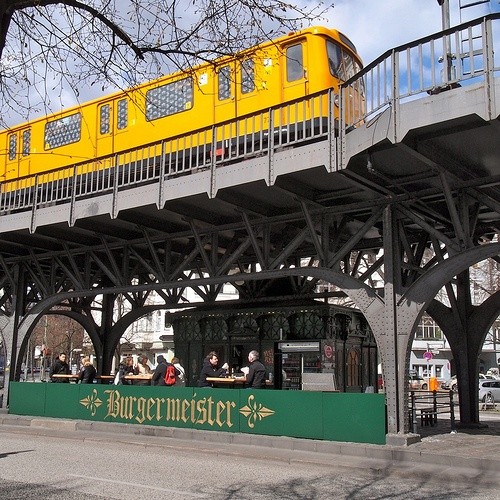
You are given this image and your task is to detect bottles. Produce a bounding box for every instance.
[285,369,300,377]
[287,355,300,367]
[291,378,299,386]
[313,369,321,373]
[366,384,374,393]
[305,355,320,367]
[289,388,298,390]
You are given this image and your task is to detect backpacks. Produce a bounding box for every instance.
[160,363,176,386]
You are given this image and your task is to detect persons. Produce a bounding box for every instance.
[49,353,71,382]
[112,353,152,385]
[242,350,266,388]
[172,358,185,383]
[152,355,180,386]
[70,353,96,383]
[199,353,229,387]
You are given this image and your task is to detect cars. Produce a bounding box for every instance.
[478,381,500,403]
[0,365,52,375]
[409,374,429,391]
[440,373,500,392]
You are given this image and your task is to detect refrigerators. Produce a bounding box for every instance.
[274,339,336,390]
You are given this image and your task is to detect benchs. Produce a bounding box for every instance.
[414,408,435,426]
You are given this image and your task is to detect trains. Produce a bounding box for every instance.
[0,25,365,204]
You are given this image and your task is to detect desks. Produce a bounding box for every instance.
[52,374,114,384]
[123,375,155,385]
[206,376,272,389]
